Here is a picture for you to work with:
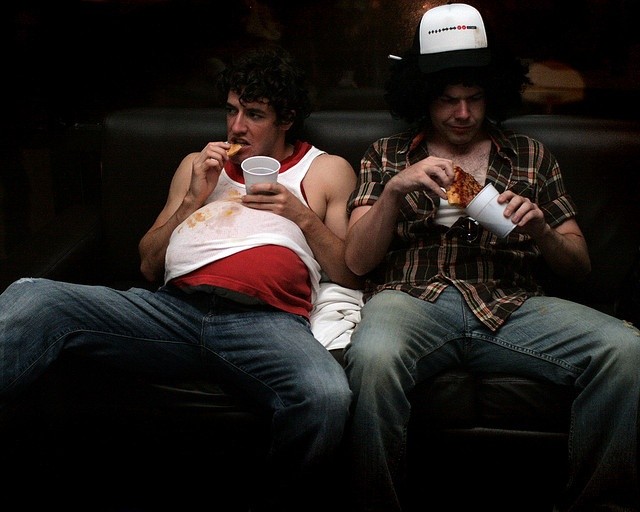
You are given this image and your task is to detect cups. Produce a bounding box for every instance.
[240,155,281,196]
[465,183,518,239]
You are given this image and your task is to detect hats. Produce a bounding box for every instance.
[412,3,491,73]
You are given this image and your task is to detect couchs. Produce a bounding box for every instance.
[1,105,640,510]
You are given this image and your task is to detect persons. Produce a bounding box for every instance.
[0,48,358,512]
[343,2,640,512]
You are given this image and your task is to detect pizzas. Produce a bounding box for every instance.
[445,166,483,206]
[226,144,244,156]
